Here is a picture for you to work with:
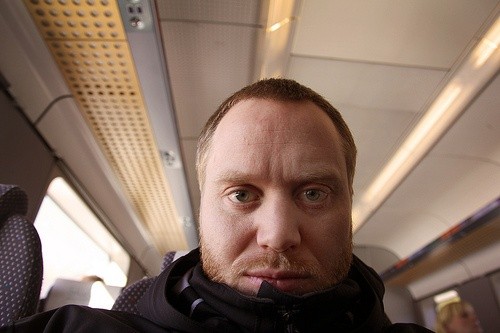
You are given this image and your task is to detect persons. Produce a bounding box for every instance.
[0,78,434,333]
[434,301,482,333]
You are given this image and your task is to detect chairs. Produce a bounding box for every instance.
[0,184,190,328]
